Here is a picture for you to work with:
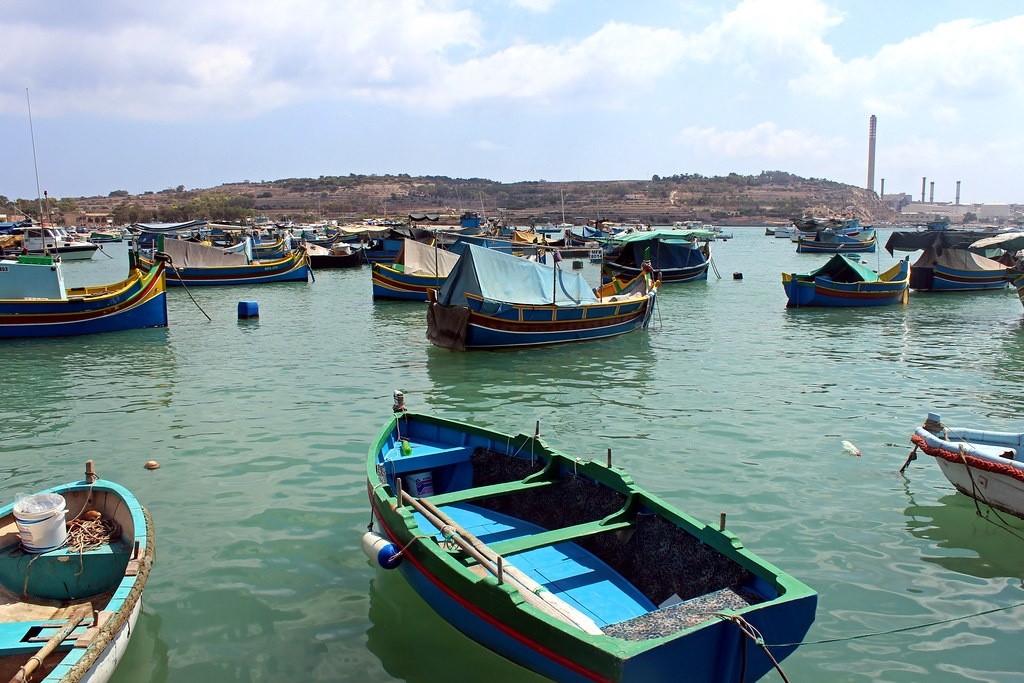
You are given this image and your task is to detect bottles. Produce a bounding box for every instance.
[402,440,413,456]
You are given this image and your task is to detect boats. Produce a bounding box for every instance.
[906,409,1024,523]
[370,236,547,302]
[887,215,1024,309]
[797,222,878,254]
[0,460,157,683]
[0,190,733,270]
[766,208,860,242]
[365,391,818,683]
[599,234,712,285]
[782,252,910,307]
[0,87,173,339]
[425,244,663,353]
[137,230,316,287]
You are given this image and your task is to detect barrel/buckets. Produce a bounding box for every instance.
[405,471,434,498]
[13,493,69,554]
